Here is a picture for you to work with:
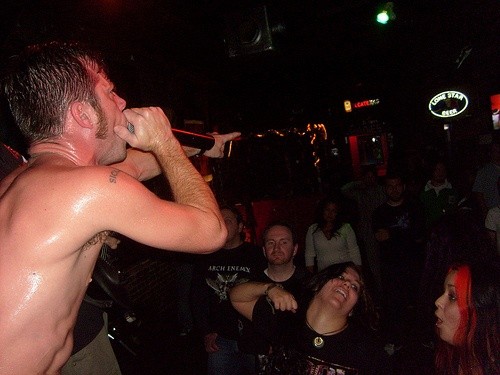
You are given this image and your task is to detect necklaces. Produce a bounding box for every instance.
[306,320,348,349]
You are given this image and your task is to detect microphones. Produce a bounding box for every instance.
[127,122,215,151]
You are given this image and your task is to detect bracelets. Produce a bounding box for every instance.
[190,141,205,157]
[264,282,283,295]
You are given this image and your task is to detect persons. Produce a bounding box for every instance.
[196,125,500,375]
[0,42,243,375]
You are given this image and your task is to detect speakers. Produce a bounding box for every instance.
[222,2,281,59]
